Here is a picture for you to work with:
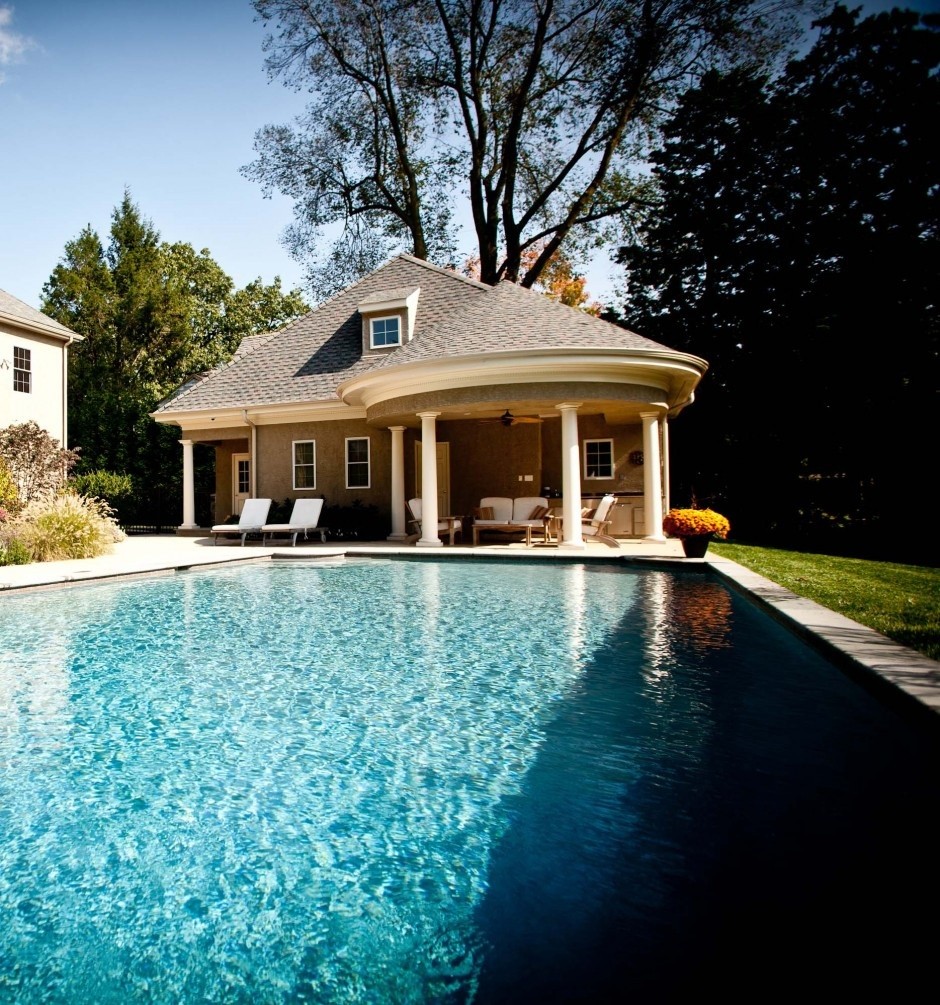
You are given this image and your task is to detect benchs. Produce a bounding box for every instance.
[471,497,551,543]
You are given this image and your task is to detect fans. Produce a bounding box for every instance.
[480,410,543,427]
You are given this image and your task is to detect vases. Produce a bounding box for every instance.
[681,535,710,558]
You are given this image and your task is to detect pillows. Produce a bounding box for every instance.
[529,505,553,520]
[581,507,597,525]
[475,507,495,519]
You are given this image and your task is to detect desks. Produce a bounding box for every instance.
[472,523,532,546]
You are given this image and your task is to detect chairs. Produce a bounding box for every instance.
[211,498,271,546]
[555,496,621,548]
[260,498,328,547]
[405,498,464,545]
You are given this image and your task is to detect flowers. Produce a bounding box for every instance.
[663,507,730,539]
